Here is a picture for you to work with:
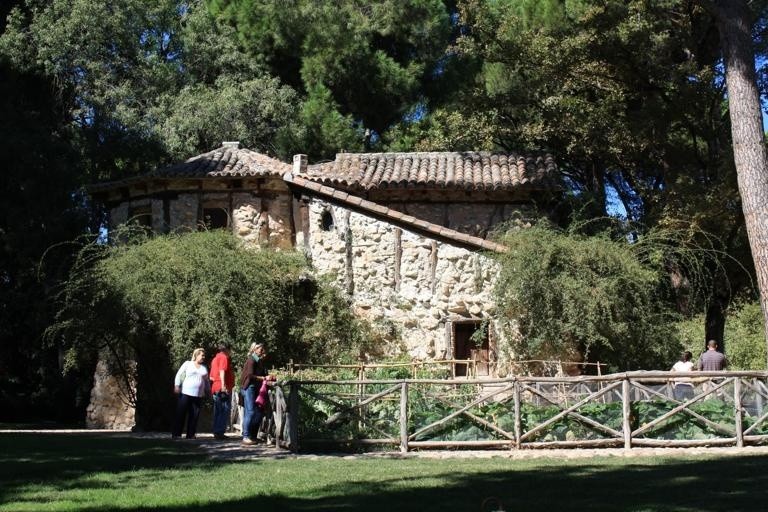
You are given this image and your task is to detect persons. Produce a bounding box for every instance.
[669,351,695,402]
[173,346,209,439]
[209,341,235,441]
[240,341,272,445]
[696,338,728,386]
